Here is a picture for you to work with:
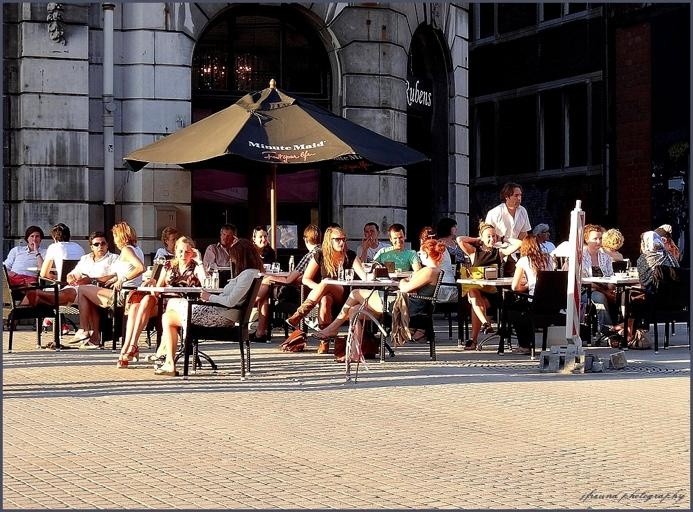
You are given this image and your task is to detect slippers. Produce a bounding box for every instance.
[311,330,338,340]
[147,354,167,363]
[153,366,179,377]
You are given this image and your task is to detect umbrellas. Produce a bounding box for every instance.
[122,78,432,258]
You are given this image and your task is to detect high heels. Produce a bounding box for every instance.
[116,344,140,369]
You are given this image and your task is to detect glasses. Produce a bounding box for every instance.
[331,237,348,243]
[90,241,107,246]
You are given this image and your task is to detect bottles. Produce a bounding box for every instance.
[288,254,295,275]
[201,267,221,290]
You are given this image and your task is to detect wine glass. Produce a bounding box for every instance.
[361,262,373,283]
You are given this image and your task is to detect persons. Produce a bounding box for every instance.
[4,179,680,375]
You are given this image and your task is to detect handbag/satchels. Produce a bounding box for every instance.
[628,328,652,349]
[278,328,308,353]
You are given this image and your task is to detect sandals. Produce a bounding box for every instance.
[463,338,478,351]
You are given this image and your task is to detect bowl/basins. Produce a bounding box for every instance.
[615,272,627,278]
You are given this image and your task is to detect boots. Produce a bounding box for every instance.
[285,299,316,327]
[316,322,332,354]
[480,321,495,335]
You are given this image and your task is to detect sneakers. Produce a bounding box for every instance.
[77,340,101,351]
[248,330,267,343]
[67,330,90,344]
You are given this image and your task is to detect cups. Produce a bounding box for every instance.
[629,267,638,279]
[262,262,281,275]
[337,267,354,282]
